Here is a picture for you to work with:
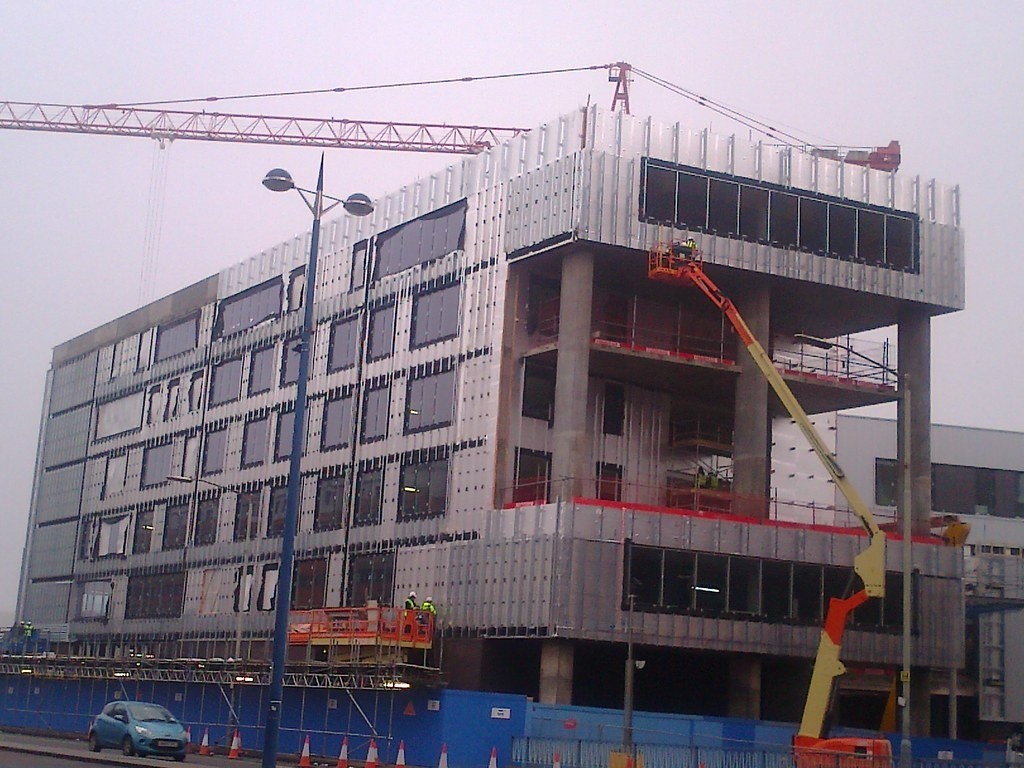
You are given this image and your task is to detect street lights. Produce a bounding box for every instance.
[790,330,912,768]
[259,168,374,768]
[166,474,254,748]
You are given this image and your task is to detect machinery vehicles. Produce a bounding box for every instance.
[649,234,893,768]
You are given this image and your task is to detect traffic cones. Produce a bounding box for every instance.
[364,738,375,768]
[237,734,243,755]
[229,729,240,760]
[393,740,407,768]
[186,726,192,754]
[200,727,210,756]
[552,749,559,767]
[437,744,448,768]
[488,748,497,768]
[296,735,310,767]
[374,743,379,766]
[334,736,348,767]
[87,722,94,740]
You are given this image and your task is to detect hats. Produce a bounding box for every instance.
[425,596,433,602]
[693,239,695,242]
[688,236,694,240]
[408,592,417,598]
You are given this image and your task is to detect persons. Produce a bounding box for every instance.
[706,471,719,489]
[403,592,421,633]
[23,622,34,640]
[695,466,706,488]
[420,596,436,634]
[686,237,698,261]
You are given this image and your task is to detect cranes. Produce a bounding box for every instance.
[0,57,898,177]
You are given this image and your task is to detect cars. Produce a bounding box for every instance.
[89,700,186,762]
